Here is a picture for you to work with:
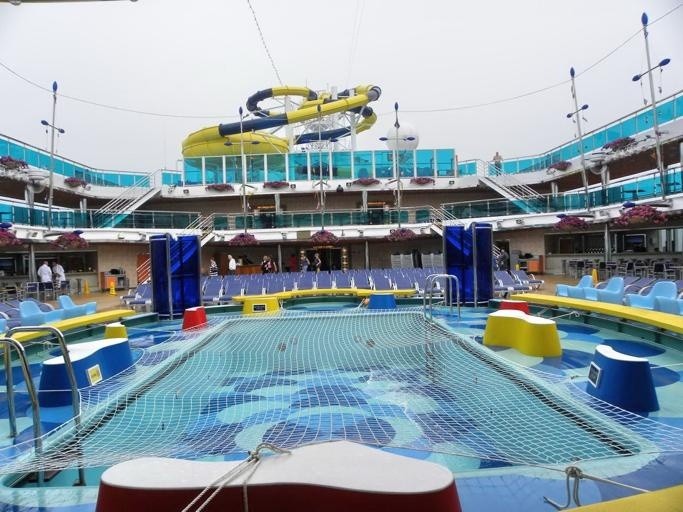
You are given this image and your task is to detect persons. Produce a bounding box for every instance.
[498,248,510,271]
[227,254,237,275]
[298,249,322,272]
[490,152,504,177]
[261,253,278,273]
[208,255,218,277]
[38,260,68,294]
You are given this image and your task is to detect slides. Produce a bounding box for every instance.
[181,85,382,172]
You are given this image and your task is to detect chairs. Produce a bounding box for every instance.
[0,278,96,335]
[120,266,445,311]
[556,257,683,317]
[493,267,545,296]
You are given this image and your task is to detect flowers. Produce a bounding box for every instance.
[206,177,436,192]
[1,229,90,252]
[228,225,419,248]
[545,137,635,175]
[65,177,87,187]
[2,155,28,171]
[553,203,669,232]
